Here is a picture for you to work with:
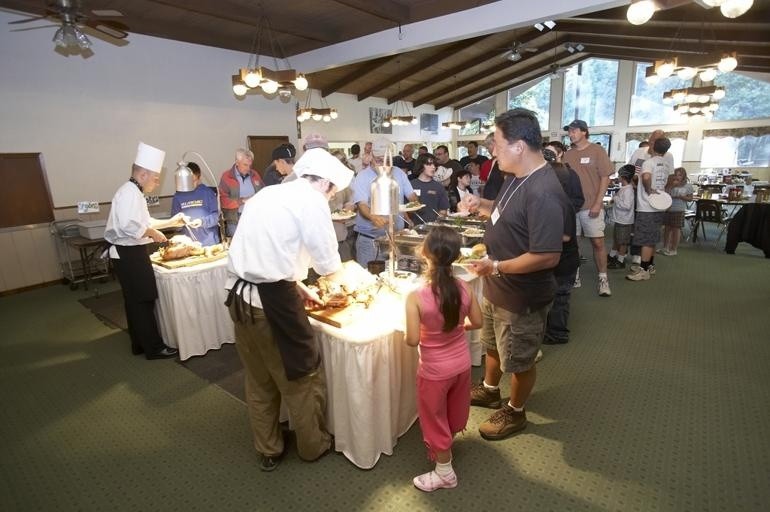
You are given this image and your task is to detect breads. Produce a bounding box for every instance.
[471,243,487,257]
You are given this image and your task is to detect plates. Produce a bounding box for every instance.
[451,212,471,218]
[648,190,673,211]
[451,247,489,266]
[399,204,426,213]
[331,212,356,221]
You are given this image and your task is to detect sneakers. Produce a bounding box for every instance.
[413,469,458,491]
[597,277,611,297]
[573,266,581,288]
[607,247,678,281]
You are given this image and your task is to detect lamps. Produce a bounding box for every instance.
[549,72,561,80]
[371,144,402,289]
[53,23,93,49]
[175,150,228,257]
[230,0,310,102]
[440,74,469,131]
[381,56,421,128]
[507,51,523,62]
[622,0,757,115]
[295,87,339,124]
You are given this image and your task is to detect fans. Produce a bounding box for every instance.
[536,29,573,80]
[5,1,129,49]
[489,28,541,63]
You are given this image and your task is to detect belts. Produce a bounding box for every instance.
[359,233,374,239]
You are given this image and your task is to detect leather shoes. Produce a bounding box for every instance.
[318,434,335,459]
[541,334,568,344]
[479,401,527,440]
[146,347,178,360]
[470,382,502,409]
[260,431,290,472]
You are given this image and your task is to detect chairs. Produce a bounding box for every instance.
[608,171,769,248]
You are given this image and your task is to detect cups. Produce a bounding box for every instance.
[698,187,714,199]
[744,187,754,197]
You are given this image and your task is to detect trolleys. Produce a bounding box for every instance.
[49,216,112,293]
[68,238,115,299]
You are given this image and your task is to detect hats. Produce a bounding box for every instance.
[370,137,394,158]
[434,166,453,182]
[563,120,587,131]
[292,147,354,192]
[134,140,166,174]
[267,146,296,167]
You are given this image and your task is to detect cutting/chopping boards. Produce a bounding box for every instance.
[150,251,225,269]
[307,283,390,328]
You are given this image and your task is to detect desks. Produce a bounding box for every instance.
[145,246,238,360]
[45,207,175,296]
[290,259,422,471]
[330,209,493,368]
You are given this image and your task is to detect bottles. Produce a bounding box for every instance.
[717,174,723,183]
[729,188,741,200]
